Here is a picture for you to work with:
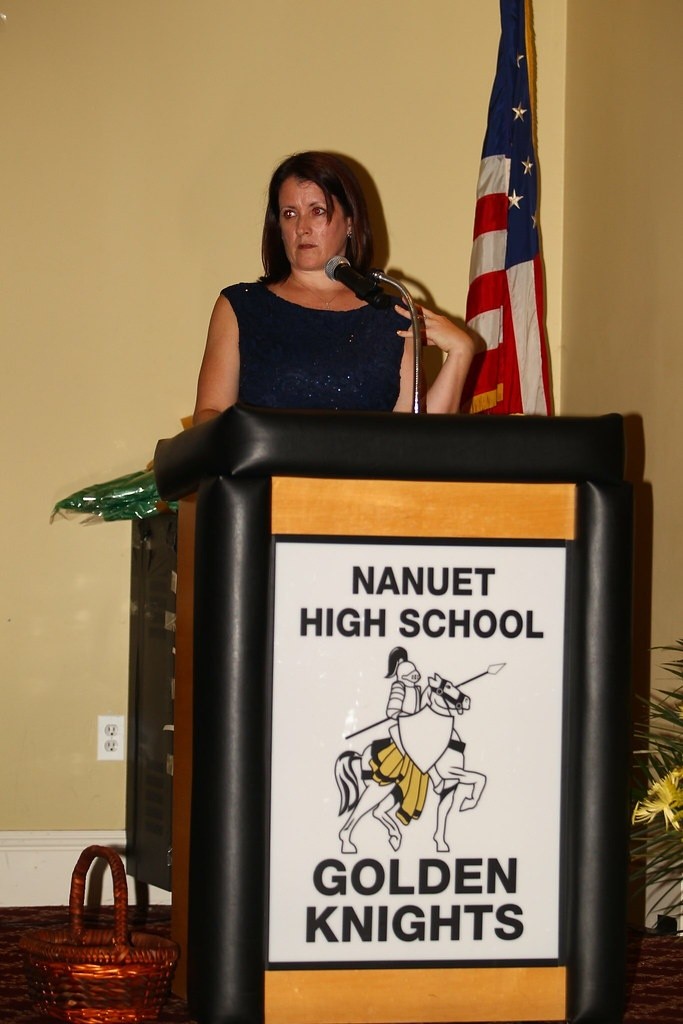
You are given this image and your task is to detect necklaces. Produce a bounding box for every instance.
[287,274,346,309]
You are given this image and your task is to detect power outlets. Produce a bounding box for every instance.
[95,714,125,762]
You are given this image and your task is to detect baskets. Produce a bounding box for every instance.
[17,844,182,1024]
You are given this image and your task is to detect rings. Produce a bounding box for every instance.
[421,314,427,324]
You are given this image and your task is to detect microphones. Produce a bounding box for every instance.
[324,256,389,310]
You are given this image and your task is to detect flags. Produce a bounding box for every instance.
[461,1,557,416]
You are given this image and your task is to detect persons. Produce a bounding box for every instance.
[163,151,475,781]
